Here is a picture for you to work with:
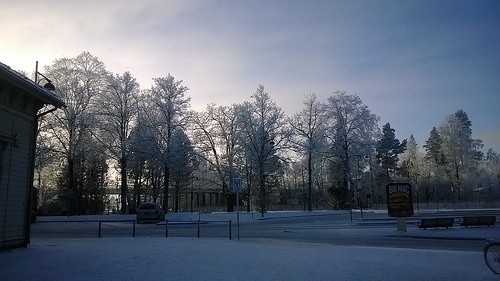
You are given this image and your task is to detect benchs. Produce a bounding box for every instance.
[417,218,454,229]
[460,216,496,228]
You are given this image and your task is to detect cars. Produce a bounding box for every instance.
[136,202,164,224]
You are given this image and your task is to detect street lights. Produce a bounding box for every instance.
[261,141,274,217]
[24,60,55,249]
[330,141,371,222]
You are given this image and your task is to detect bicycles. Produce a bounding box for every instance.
[484,239,500,275]
